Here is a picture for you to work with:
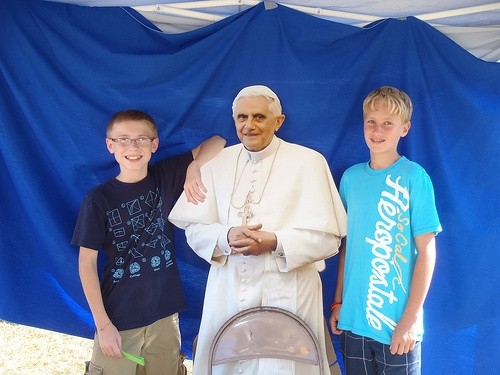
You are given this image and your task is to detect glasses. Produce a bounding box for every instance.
[109,137,154,145]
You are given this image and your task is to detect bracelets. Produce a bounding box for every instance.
[96,320,112,332]
[331,301,342,310]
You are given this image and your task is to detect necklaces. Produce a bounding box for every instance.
[231,138,280,226]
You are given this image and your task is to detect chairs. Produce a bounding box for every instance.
[207,306,324,375]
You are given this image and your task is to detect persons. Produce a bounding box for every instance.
[69,110,226,375]
[168,85,349,375]
[329,87,443,375]
